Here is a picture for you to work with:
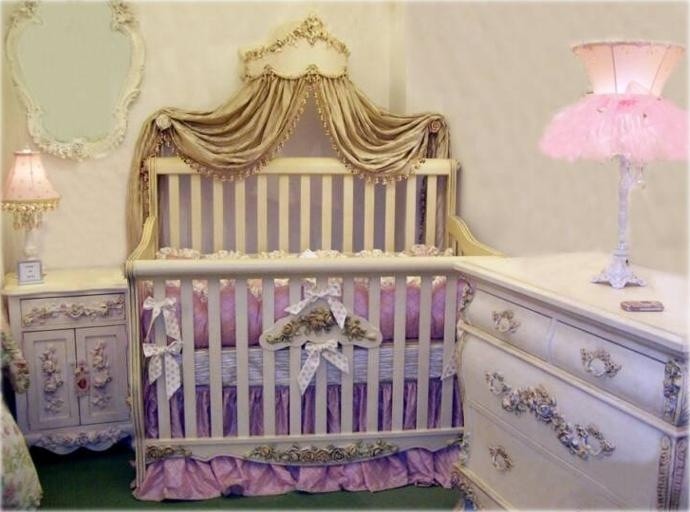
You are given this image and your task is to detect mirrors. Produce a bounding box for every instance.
[5,0,146,163]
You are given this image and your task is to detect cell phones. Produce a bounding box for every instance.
[622,300,665,312]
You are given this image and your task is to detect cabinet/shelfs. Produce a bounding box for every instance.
[0,266,135,456]
[448,254,690,511]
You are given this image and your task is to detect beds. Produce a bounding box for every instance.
[120,155,507,499]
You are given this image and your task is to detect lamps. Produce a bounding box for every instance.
[537,39,689,293]
[0,140,62,276]
